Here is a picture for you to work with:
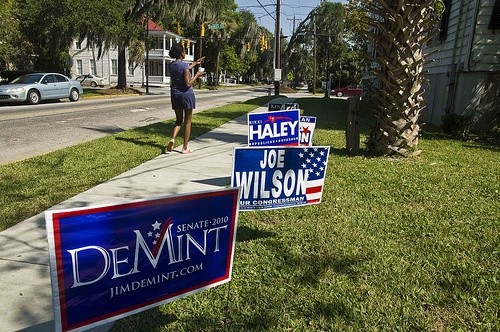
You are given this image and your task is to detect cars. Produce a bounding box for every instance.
[71,74,109,88]
[331,83,364,97]
[0,71,84,105]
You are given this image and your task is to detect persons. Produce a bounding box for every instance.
[167,43,206,154]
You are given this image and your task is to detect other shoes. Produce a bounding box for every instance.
[166,139,175,152]
[182,149,193,153]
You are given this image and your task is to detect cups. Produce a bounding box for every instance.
[198,67,205,72]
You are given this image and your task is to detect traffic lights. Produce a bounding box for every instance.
[263,40,267,49]
[260,36,265,46]
